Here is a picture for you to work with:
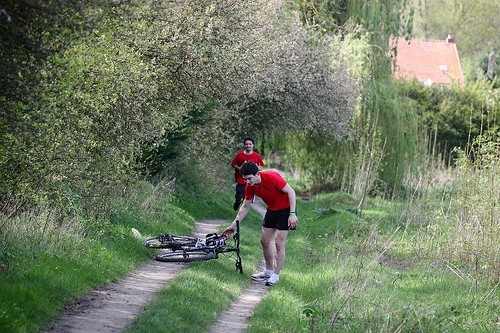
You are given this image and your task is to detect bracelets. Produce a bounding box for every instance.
[289,212,297,216]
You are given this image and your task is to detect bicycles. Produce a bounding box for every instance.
[144,220,244,274]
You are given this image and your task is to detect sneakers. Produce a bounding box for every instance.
[234,202,240,211]
[251,195,256,203]
[251,270,280,286]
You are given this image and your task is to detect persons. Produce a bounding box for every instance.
[221,162,299,287]
[230,138,265,211]
